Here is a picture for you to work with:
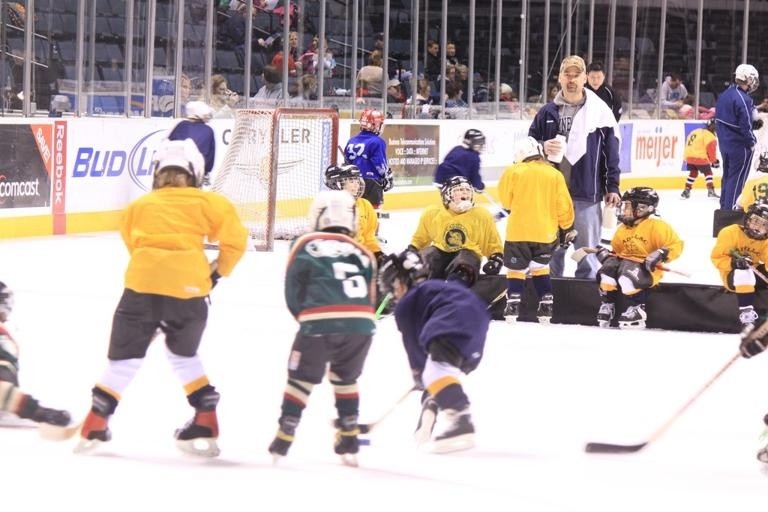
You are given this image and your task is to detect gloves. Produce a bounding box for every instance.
[559,225,576,248]
[596,245,609,263]
[644,249,667,272]
[483,254,504,275]
[732,256,751,269]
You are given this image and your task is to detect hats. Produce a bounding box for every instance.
[560,55,586,76]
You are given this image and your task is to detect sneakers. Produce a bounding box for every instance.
[708,191,719,197]
[435,415,472,441]
[413,400,438,434]
[619,303,646,321]
[597,303,616,320]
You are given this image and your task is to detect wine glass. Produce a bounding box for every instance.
[4,77,14,114]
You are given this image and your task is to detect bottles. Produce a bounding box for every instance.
[601,199,618,229]
[751,106,759,123]
[402,96,413,120]
[547,133,568,165]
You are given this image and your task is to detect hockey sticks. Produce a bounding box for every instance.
[588,352,742,454]
[570,246,692,277]
[332,387,416,434]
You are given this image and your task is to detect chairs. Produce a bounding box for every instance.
[0,0,768,115]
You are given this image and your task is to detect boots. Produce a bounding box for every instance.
[174,393,220,439]
[333,418,359,454]
[502,294,521,315]
[536,293,553,317]
[270,417,297,457]
[75,390,118,442]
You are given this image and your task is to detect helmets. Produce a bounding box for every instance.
[186,101,212,120]
[378,250,424,303]
[307,164,366,235]
[465,129,486,153]
[359,109,384,135]
[616,187,658,227]
[441,177,476,211]
[151,138,205,187]
[744,199,767,239]
[513,136,544,164]
[736,63,760,91]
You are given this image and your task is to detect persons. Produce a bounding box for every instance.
[7,43,67,110]
[538,51,623,122]
[433,129,486,193]
[344,109,393,209]
[356,32,520,119]
[323,164,389,315]
[596,186,684,330]
[528,55,621,280]
[75,138,251,440]
[267,204,377,454]
[752,64,768,112]
[713,63,764,210]
[660,71,717,121]
[711,151,768,341]
[0,282,73,427]
[202,0,336,77]
[397,175,504,291]
[680,120,721,199]
[378,260,490,440]
[498,136,574,325]
[740,314,768,472]
[180,72,318,114]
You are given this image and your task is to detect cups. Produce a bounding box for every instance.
[225,90,240,106]
[421,103,429,114]
